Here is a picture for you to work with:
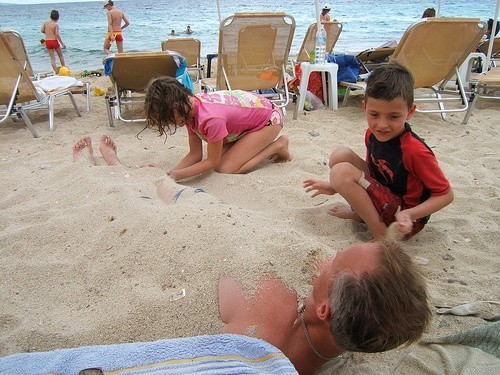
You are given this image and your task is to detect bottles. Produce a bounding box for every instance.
[315,25,328,64]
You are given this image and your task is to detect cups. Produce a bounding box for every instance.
[59,66,70,76]
[95,88,105,96]
[310,49,315,63]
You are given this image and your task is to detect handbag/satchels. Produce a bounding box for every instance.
[293,65,324,104]
[297,86,325,111]
[319,52,360,84]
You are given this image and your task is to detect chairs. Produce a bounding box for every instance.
[199,11,295,116]
[287,20,342,97]
[160,38,206,81]
[103,51,195,128]
[340,17,488,121]
[356,46,395,74]
[462,71,500,124]
[0,29,91,137]
[1,333,302,375]
[231,25,281,79]
[473,37,500,68]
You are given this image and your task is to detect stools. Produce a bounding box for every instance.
[457,52,488,90]
[207,54,229,79]
[292,61,339,121]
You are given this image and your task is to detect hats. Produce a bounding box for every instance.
[103,0,113,9]
[322,3,331,9]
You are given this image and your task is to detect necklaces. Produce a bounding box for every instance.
[297,305,337,360]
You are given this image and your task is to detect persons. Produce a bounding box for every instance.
[302,65,453,240]
[171,30,175,35]
[186,26,190,33]
[423,8,435,18]
[320,3,337,52]
[137,76,291,181]
[40,39,45,44]
[103,0,129,55]
[471,19,500,52]
[41,10,66,74]
[72,135,431,375]
